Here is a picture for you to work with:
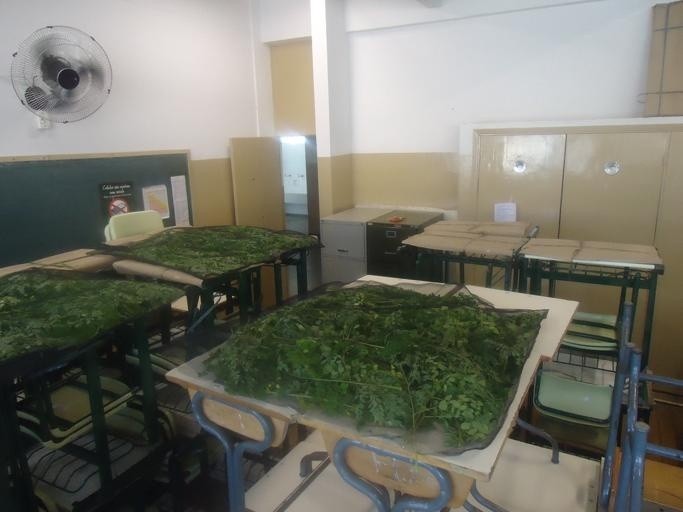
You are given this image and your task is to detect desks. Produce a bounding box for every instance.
[2,217,667,512]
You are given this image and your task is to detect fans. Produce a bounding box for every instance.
[7,26,113,132]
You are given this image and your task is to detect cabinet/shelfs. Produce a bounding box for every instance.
[319,205,444,282]
